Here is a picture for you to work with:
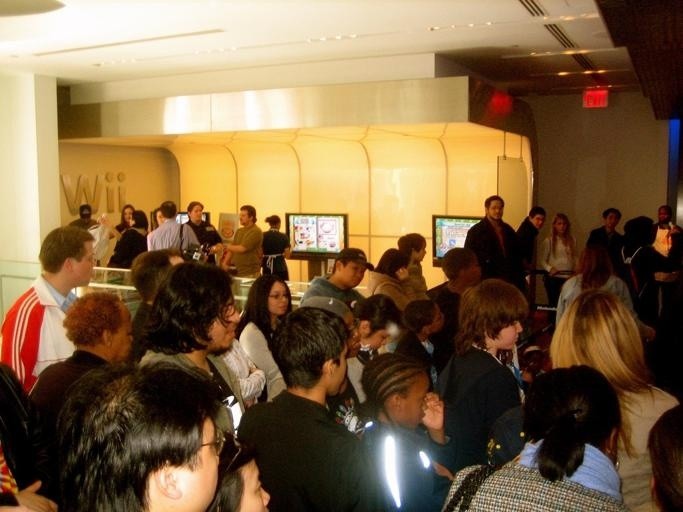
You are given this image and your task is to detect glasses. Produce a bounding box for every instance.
[200,428,225,454]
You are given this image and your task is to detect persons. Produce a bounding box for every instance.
[439,365,636,512]
[53,355,226,511]
[360,352,458,511]
[206,430,270,511]
[547,291,679,511]
[235,307,390,511]
[0,360,58,511]
[642,402,682,511]
[0,196,682,469]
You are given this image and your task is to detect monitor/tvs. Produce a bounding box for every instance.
[431,214,480,259]
[284,212,347,256]
[177,211,209,224]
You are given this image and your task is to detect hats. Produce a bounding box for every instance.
[336,249,374,272]
[79,204,91,217]
